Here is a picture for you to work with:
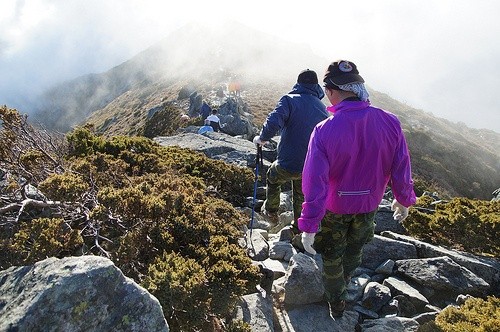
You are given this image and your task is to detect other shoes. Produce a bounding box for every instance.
[290,226,302,237]
[328,300,345,320]
[260,209,281,225]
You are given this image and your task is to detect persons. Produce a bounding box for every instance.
[297,60,417,320]
[253,68,329,237]
[198,108,221,136]
[228,79,241,96]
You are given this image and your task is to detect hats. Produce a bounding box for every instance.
[297,68,319,85]
[322,60,365,85]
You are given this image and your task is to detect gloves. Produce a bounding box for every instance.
[391,198,409,223]
[301,231,317,255]
[253,136,268,150]
